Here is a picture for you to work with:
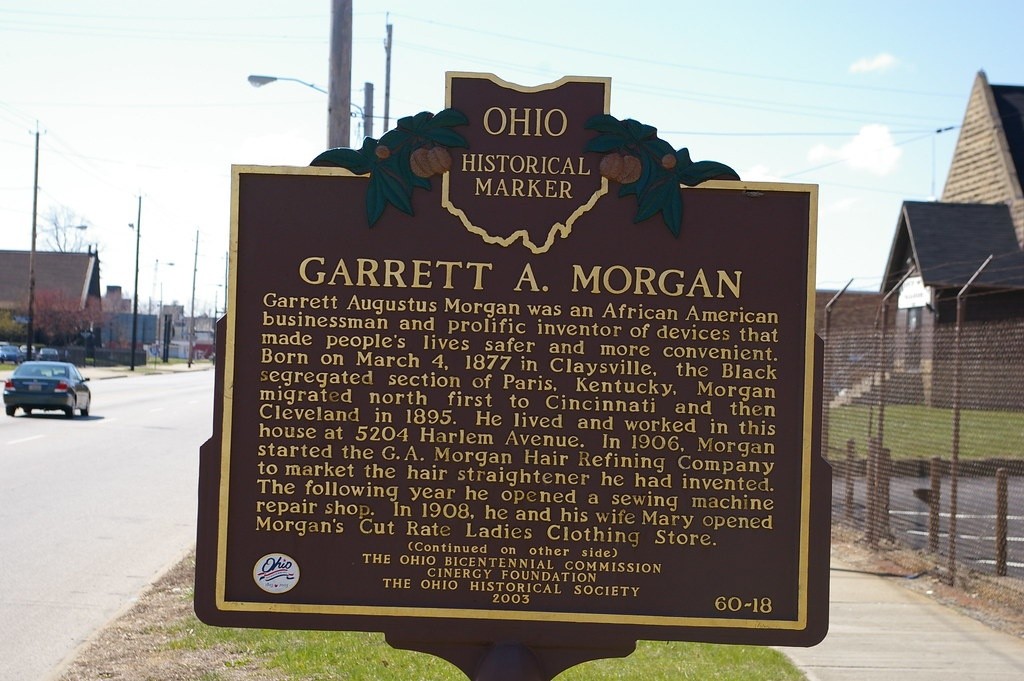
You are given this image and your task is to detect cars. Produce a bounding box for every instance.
[40,348,59,361]
[20,345,36,361]
[0,341,24,365]
[3,361,91,418]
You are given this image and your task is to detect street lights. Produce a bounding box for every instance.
[249,74,375,152]
[26,225,88,362]
[213,284,223,363]
[151,259,174,312]
